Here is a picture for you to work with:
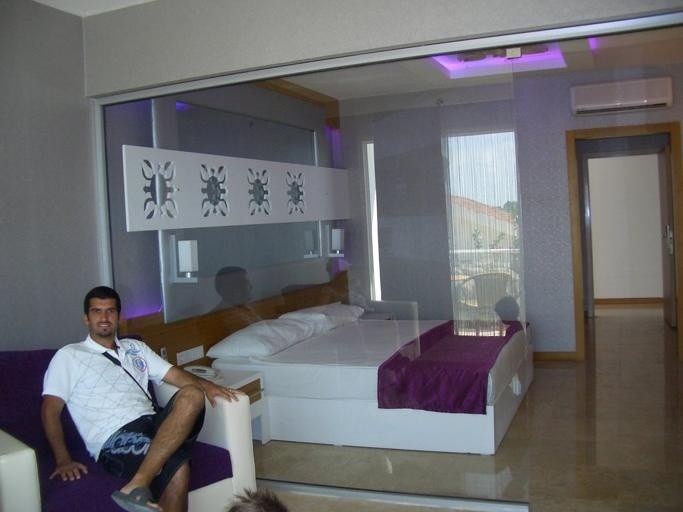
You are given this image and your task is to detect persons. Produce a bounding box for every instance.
[40,286,245,512]
[227,487,289,512]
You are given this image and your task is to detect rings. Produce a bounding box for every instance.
[229,389,232,393]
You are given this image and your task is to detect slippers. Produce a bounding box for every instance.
[112,487,162,511]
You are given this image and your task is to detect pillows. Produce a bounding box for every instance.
[205,318,316,359]
[278,301,365,333]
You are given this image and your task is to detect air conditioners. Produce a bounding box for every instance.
[571,76,672,116]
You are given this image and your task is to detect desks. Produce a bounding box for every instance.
[207,367,273,450]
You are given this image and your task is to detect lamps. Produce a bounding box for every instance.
[176,237,200,279]
[331,227,346,254]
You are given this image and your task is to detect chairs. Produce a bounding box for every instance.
[453,272,521,337]
[0,342,258,512]
[359,299,421,361]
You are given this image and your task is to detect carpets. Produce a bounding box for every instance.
[254,475,530,512]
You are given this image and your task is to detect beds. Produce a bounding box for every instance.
[211,316,535,458]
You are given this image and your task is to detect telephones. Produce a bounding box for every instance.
[184,365,217,379]
